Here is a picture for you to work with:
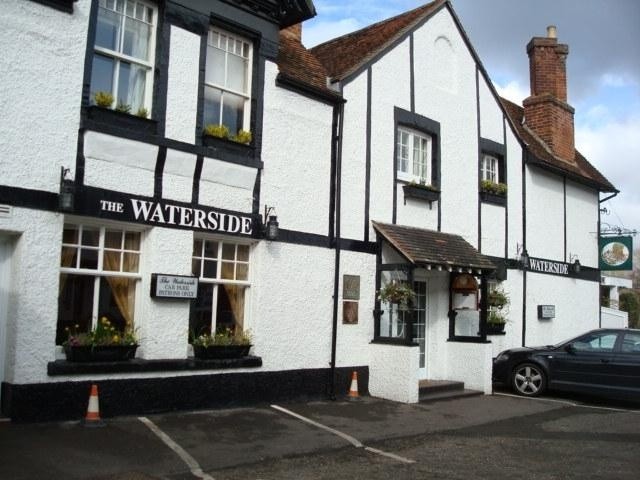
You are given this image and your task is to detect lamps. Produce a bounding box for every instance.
[60,167,75,209]
[517,244,529,269]
[569,253,581,275]
[262,204,278,240]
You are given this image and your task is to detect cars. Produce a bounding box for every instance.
[490,327,639,408]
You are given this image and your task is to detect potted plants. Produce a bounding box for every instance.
[486,287,510,311]
[401,179,442,201]
[63,317,139,360]
[486,310,511,330]
[87,92,159,135]
[202,123,255,158]
[191,325,253,360]
[478,180,508,205]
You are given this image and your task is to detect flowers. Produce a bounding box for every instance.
[375,278,417,305]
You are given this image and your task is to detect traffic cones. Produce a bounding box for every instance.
[345,371,364,402]
[81,385,109,429]
